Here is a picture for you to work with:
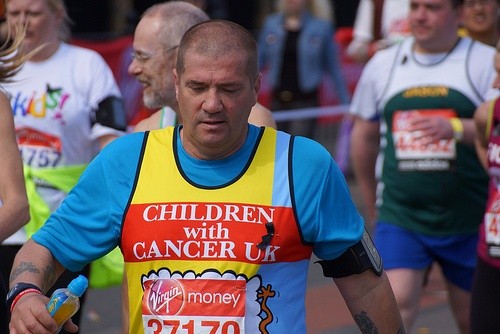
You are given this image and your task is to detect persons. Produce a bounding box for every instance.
[6,19,404,334]
[0,0,128,334]
[127,2,353,141]
[348,0,500,334]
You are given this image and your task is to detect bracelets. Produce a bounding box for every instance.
[447,116,464,144]
[9,289,42,314]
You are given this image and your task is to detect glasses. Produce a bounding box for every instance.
[129,45,180,65]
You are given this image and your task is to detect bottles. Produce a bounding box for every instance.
[46,275,88,334]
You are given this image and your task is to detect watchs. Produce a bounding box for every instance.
[6,283,42,307]
